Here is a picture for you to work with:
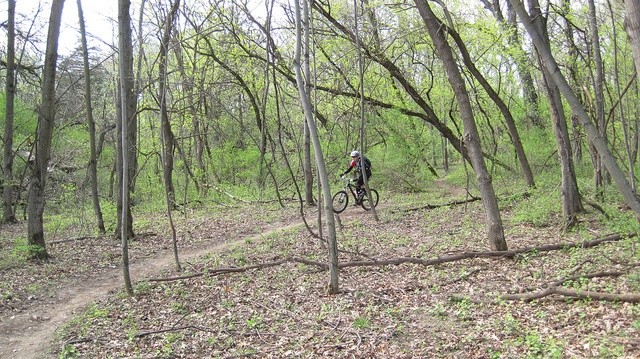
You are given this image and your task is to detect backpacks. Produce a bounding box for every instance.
[360,156,371,167]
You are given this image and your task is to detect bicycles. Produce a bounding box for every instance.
[332,175,379,213]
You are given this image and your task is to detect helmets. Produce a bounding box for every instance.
[351,151,360,157]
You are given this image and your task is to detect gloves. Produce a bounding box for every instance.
[340,174,344,177]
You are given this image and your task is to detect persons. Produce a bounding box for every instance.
[339,150,371,206]
